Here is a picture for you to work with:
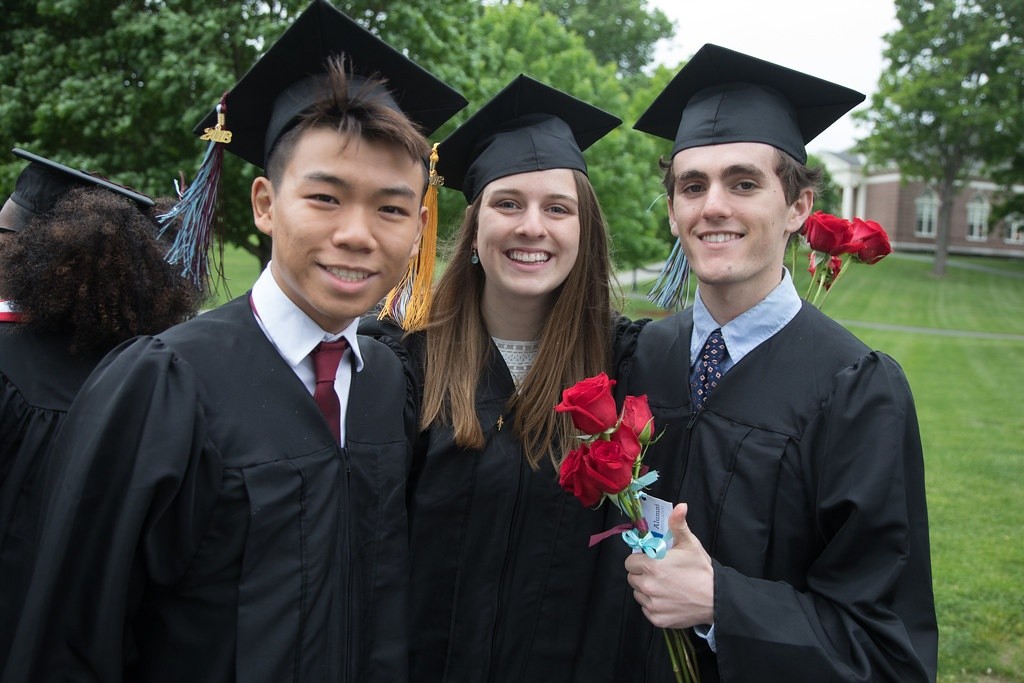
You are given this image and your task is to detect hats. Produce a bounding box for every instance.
[632,43,867,311]
[158,0,470,290]
[376,74,624,331]
[0,149,155,232]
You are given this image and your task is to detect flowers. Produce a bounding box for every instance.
[553,372,703,683]
[802,209,895,310]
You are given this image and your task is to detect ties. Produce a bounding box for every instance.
[250,293,348,450]
[691,328,728,410]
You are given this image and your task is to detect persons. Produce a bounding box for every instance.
[0,74,433,683]
[610,83,937,683]
[374,123,652,683]
[0,146,201,683]
